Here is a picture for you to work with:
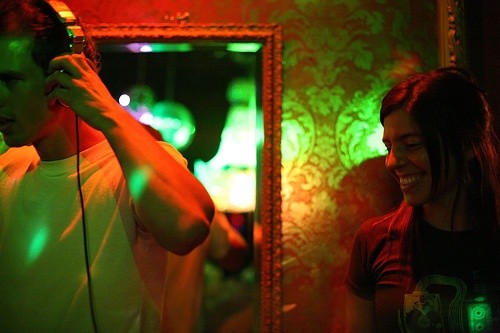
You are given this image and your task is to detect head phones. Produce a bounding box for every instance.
[37,0,91,110]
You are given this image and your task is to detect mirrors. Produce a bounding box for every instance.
[71,18,288,333]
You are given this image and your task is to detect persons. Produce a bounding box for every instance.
[341,66,500,332]
[89,39,249,333]
[0,0,216,333]
